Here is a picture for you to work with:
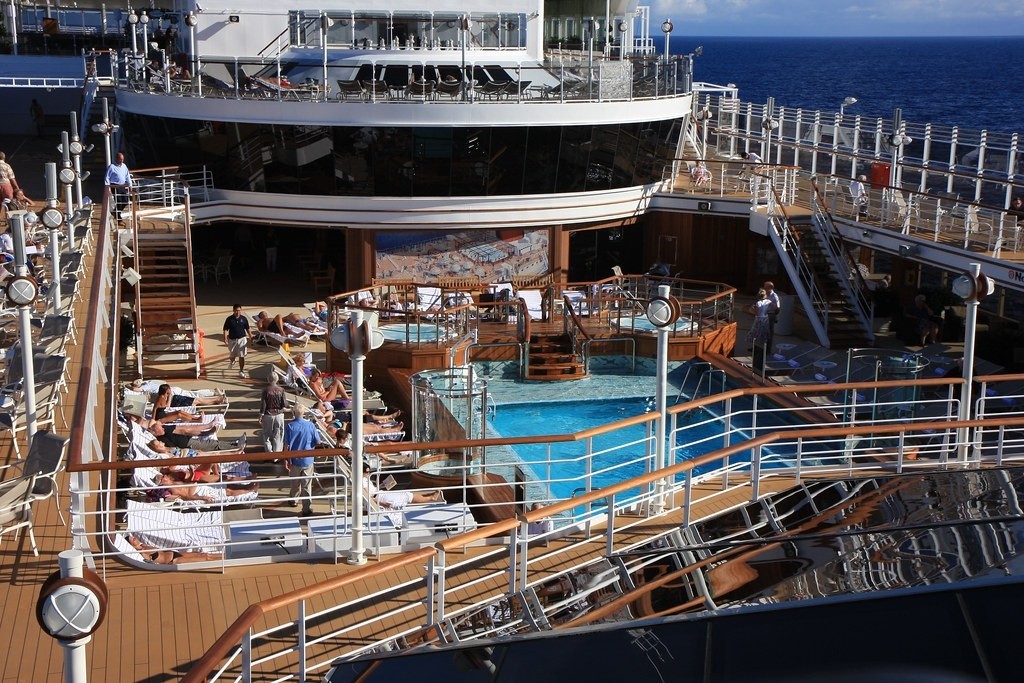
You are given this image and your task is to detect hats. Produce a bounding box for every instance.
[13,189,23,197]
[25,217,34,225]
[756,289,767,299]
[858,174,867,180]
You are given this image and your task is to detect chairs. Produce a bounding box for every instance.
[0,46,1024,577]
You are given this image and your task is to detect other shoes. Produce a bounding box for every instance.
[119,221,125,226]
[239,371,247,378]
[237,432,247,450]
[288,501,298,507]
[227,360,237,368]
[111,211,122,219]
[300,336,308,348]
[302,509,313,515]
[747,347,753,352]
[430,490,440,501]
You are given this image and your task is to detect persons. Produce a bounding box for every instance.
[360,292,396,314]
[0,151,36,278]
[283,312,326,333]
[155,25,192,79]
[747,281,780,354]
[125,536,225,564]
[879,275,892,289]
[455,298,478,314]
[258,311,305,338]
[738,152,764,183]
[914,295,938,345]
[285,403,321,516]
[224,304,251,379]
[689,160,712,186]
[259,372,289,463]
[105,153,132,226]
[249,75,300,100]
[603,289,612,294]
[499,288,518,316]
[849,175,868,216]
[1008,197,1024,221]
[314,302,346,322]
[943,358,976,392]
[125,380,259,502]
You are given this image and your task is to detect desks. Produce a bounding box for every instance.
[855,273,886,294]
[814,361,837,373]
[777,344,795,353]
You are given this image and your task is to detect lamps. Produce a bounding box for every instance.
[898,244,920,255]
[121,265,142,287]
[694,46,704,57]
[840,97,858,120]
[224,516,303,557]
[118,393,149,416]
[400,501,478,546]
[307,514,398,554]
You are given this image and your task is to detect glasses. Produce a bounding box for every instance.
[365,471,370,473]
